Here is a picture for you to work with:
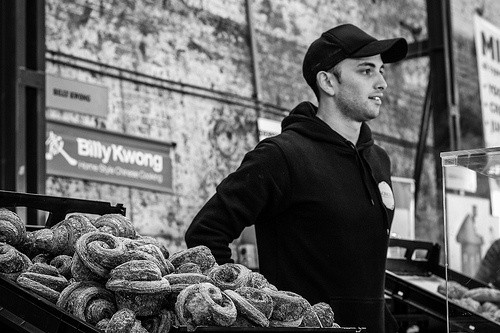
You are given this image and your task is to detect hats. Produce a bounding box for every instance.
[302,24,408,85]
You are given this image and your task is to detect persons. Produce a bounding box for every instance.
[476,238,500,290]
[184,24,408,333]
